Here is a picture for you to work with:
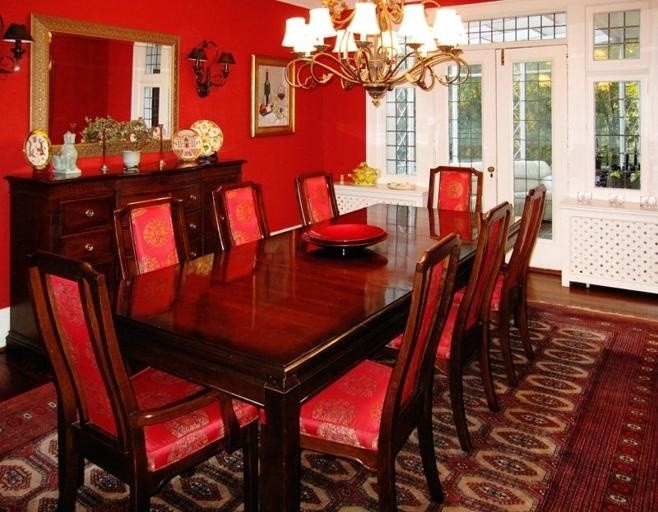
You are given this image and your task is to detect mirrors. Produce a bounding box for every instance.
[26,13,188,163]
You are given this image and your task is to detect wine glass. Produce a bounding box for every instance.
[277,85,286,114]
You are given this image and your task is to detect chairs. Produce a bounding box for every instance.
[259,231,462,512]
[113,195,191,280]
[295,171,341,226]
[427,165,484,214]
[210,180,271,251]
[23,249,260,511]
[384,200,514,454]
[464,182,552,390]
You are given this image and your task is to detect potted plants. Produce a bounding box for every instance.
[109,116,158,171]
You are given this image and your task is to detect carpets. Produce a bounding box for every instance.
[0,296,658,512]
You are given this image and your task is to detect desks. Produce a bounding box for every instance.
[110,203,521,511]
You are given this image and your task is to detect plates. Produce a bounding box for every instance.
[190,120,224,159]
[388,183,417,191]
[171,128,205,163]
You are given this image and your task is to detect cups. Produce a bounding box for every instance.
[575,191,657,212]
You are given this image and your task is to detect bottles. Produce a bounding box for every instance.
[264,72,272,106]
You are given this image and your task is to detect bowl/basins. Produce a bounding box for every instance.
[301,224,388,255]
[303,248,387,271]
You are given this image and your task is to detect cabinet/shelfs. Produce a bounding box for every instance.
[3,159,252,379]
[561,199,657,293]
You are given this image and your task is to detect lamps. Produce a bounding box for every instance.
[278,0,476,109]
[184,36,236,102]
[0,16,30,78]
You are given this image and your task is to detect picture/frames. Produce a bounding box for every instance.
[248,50,298,141]
